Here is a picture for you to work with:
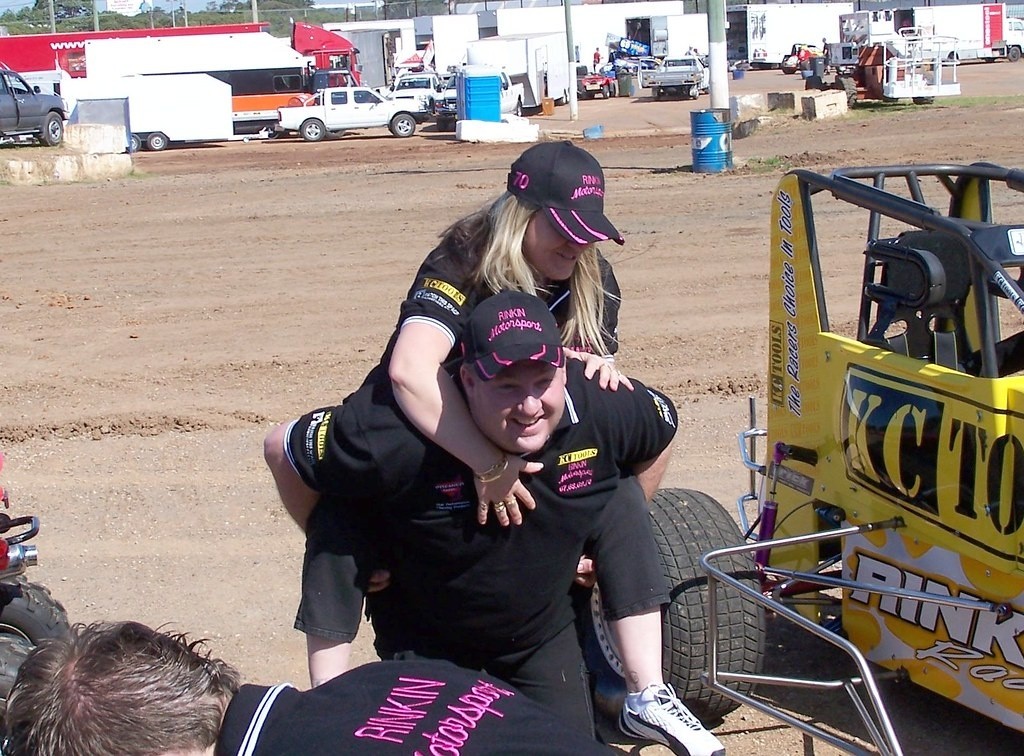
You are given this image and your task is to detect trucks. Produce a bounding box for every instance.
[893,2,1024,64]
[726,3,895,75]
[85,31,361,139]
[0,19,364,140]
[20,68,234,153]
[436,32,568,132]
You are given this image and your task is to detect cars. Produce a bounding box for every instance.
[574,61,617,100]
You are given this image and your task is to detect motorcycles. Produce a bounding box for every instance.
[0,464,72,739]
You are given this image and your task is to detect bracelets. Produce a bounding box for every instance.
[474,451,509,483]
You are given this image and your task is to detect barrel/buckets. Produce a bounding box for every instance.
[618,73,631,97]
[583,125,603,140]
[733,70,743,79]
[691,109,733,173]
[543,98,554,116]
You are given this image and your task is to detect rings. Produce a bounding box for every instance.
[506,497,516,508]
[495,501,505,512]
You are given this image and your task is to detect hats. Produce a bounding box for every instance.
[460,290,564,381]
[507,141,626,246]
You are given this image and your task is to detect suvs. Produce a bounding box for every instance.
[386,63,444,123]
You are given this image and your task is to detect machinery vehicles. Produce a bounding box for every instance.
[803,26,962,109]
[573,160,1024,756]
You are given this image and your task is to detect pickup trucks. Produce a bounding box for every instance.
[637,55,709,100]
[277,86,428,141]
[0,66,70,148]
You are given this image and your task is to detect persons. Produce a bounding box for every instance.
[291,140,732,756]
[261,293,683,753]
[575,45,580,63]
[592,47,605,71]
[0,618,636,756]
[822,38,830,75]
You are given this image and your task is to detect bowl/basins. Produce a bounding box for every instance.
[782,67,798,74]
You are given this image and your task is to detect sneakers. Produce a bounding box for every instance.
[618,684,727,756]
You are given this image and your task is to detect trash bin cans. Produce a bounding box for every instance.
[448,66,502,124]
[689,108,734,173]
[809,56,827,78]
